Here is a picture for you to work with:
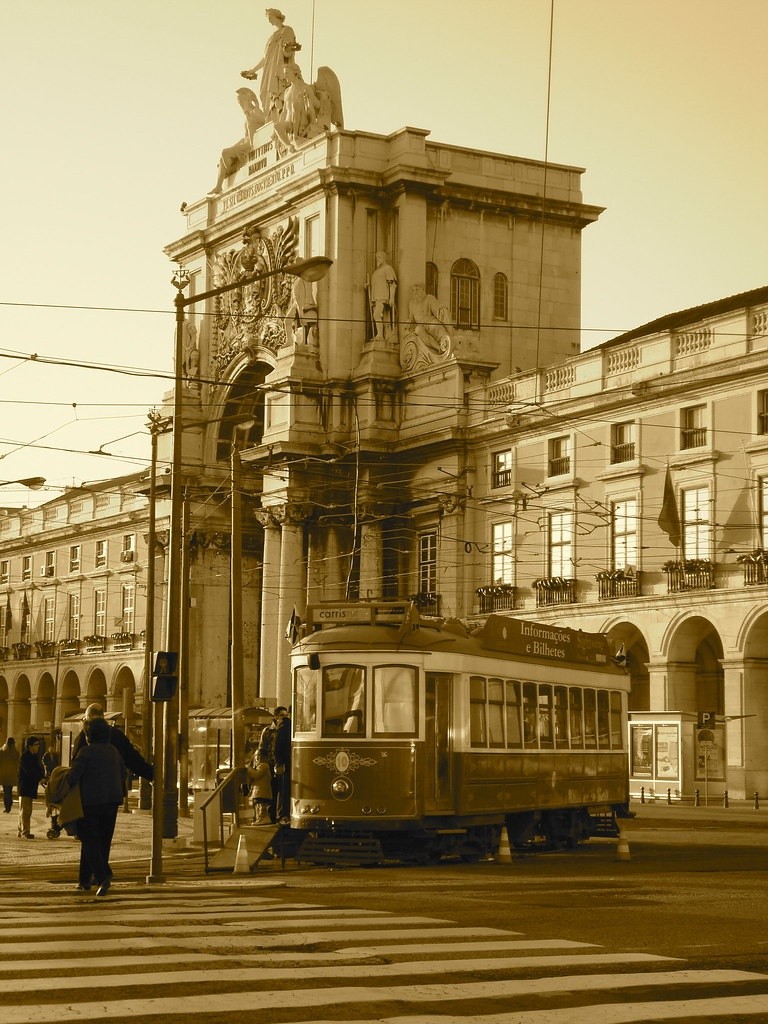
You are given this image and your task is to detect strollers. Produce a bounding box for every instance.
[40,779,82,842]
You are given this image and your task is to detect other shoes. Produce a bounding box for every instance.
[73,884,91,890]
[277,816,290,826]
[95,880,110,896]
[251,818,271,826]
[21,834,34,839]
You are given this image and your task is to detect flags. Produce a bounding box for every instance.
[20,593,30,637]
[658,470,681,547]
[283,604,303,646]
[5,596,13,637]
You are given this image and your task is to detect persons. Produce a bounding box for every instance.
[405,280,450,353]
[42,744,60,777]
[258,706,289,824]
[68,717,128,896]
[71,704,155,886]
[364,251,397,342]
[281,257,317,348]
[0,736,19,814]
[206,87,266,195]
[245,748,272,826]
[247,7,296,114]
[17,736,47,838]
[274,64,320,153]
[173,316,198,389]
[274,705,290,827]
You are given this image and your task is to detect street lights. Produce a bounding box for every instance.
[161,253,334,829]
[138,414,259,811]
[0,477,46,490]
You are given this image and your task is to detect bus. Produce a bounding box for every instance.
[283,598,635,863]
[58,708,274,798]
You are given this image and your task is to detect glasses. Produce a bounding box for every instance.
[32,743,40,745]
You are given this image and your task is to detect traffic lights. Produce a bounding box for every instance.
[146,651,178,701]
